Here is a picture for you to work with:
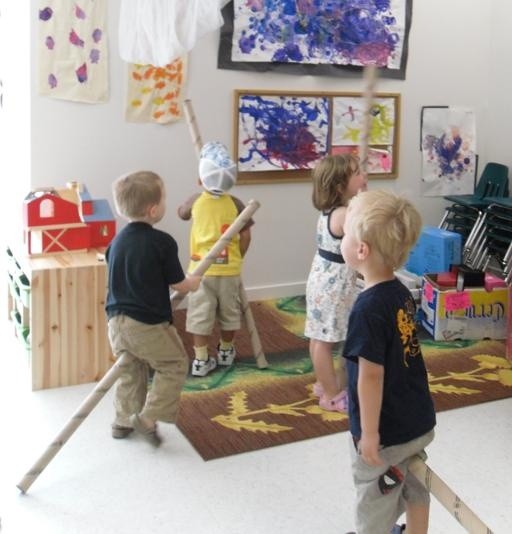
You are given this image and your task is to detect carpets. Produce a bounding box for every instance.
[147,294,512,462]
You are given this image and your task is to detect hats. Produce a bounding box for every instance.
[199,140,237,195]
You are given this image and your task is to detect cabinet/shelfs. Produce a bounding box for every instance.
[7,244,116,391]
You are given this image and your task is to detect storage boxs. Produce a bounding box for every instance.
[418,273,510,342]
[404,226,462,276]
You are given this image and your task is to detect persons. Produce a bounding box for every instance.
[178,140,256,378]
[305,154,368,414]
[340,190,437,534]
[104,171,202,449]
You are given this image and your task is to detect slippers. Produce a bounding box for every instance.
[112,424,134,438]
[130,413,161,448]
[313,383,324,397]
[319,390,349,415]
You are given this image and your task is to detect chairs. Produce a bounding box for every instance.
[438,162,512,282]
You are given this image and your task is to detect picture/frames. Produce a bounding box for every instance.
[230,89,401,186]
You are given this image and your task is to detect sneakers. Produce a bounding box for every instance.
[191,355,218,376]
[216,344,237,366]
